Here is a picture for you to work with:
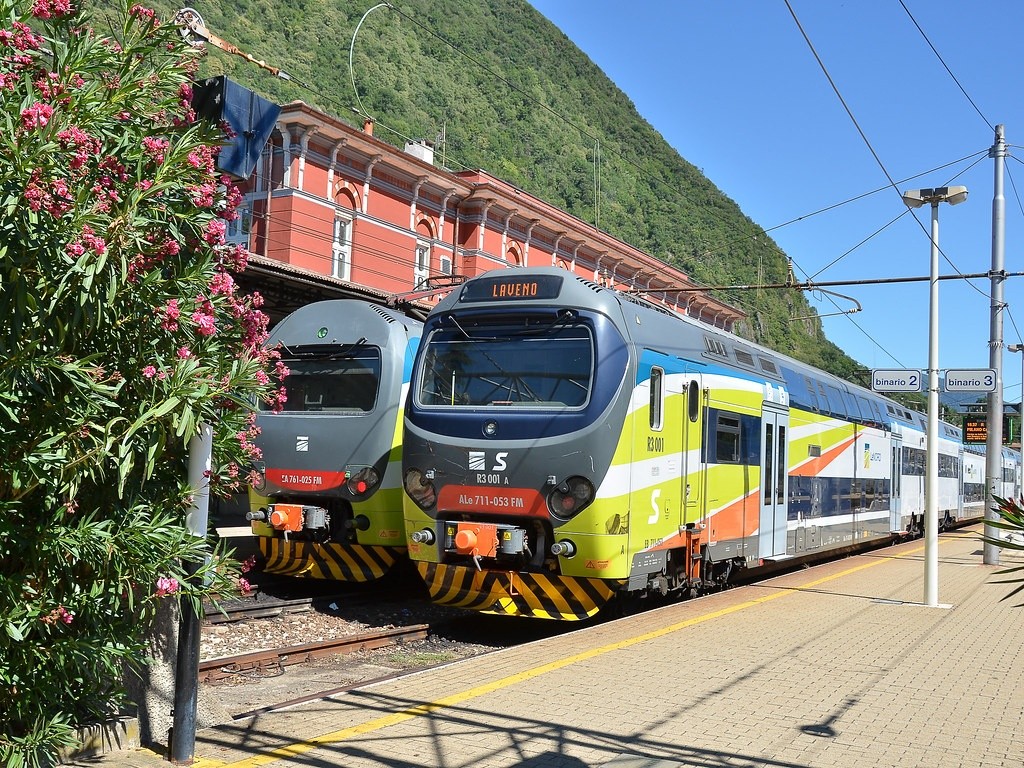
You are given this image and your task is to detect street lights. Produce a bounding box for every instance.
[902,184,971,607]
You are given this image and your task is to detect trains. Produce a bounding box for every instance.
[245,266,1021,621]
